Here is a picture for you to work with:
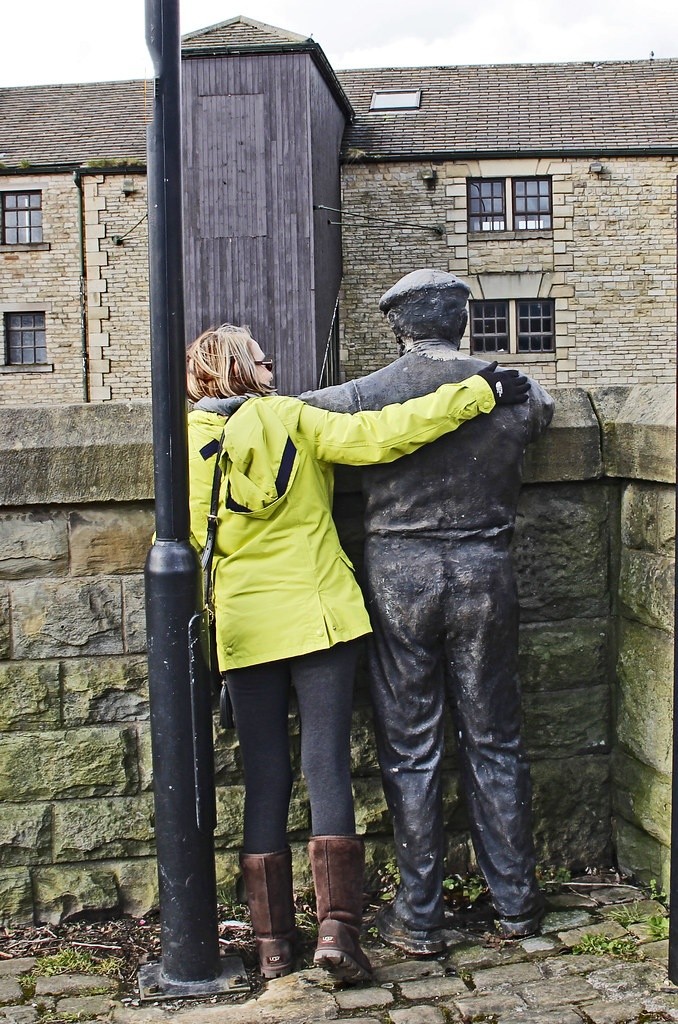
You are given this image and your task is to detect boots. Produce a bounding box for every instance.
[239,846,301,979]
[307,834,374,983]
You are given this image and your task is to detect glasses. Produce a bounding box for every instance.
[254,359,273,371]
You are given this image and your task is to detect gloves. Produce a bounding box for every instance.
[476,360,531,405]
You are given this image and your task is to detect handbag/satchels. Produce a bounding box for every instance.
[207,613,235,730]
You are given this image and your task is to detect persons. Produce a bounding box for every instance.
[302,269,556,956]
[180,321,533,988]
